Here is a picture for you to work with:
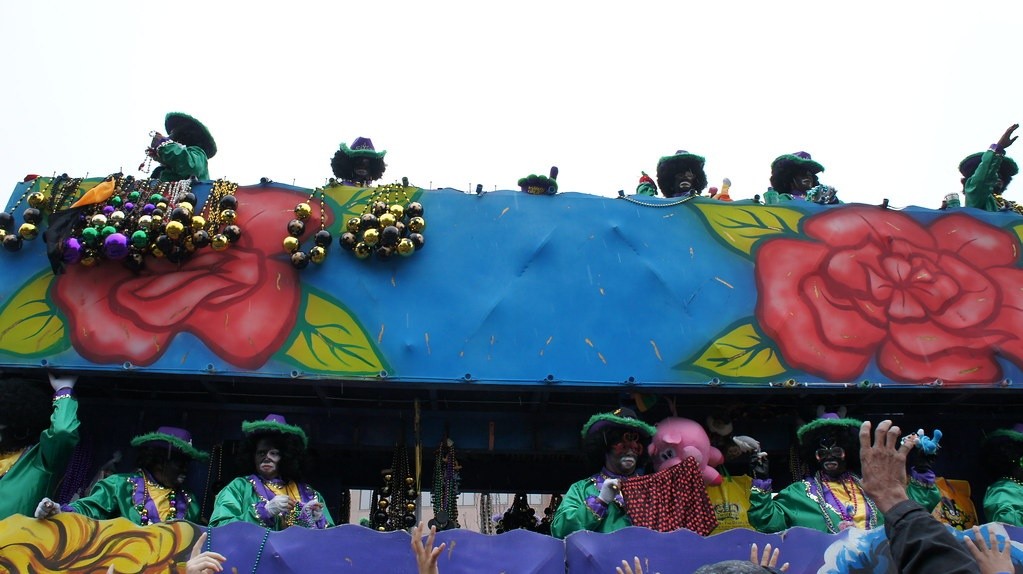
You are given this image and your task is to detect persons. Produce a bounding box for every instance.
[150,112,216,181]
[331,137,386,187]
[981,429,1023,527]
[208,414,335,531]
[747,414,942,534]
[551,407,657,539]
[963,525,1015,574]
[859,420,980,574]
[616,543,789,574]
[0,366,81,522]
[959,123,1023,214]
[763,152,844,204]
[34,427,211,525]
[636,150,707,197]
[186,531,226,574]
[410,521,445,574]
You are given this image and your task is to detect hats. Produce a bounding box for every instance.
[658,149,706,198]
[241,413,308,451]
[983,423,1023,443]
[581,406,656,443]
[771,151,824,194]
[797,413,863,462]
[959,144,1018,178]
[164,113,217,159]
[131,426,210,460]
[330,137,388,181]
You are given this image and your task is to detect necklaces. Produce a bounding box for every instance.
[201,444,222,525]
[480,493,493,535]
[53,426,96,505]
[814,471,877,534]
[263,482,296,502]
[139,470,175,526]
[138,131,158,173]
[430,435,462,531]
[789,439,812,482]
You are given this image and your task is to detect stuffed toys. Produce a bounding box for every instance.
[648,417,724,486]
